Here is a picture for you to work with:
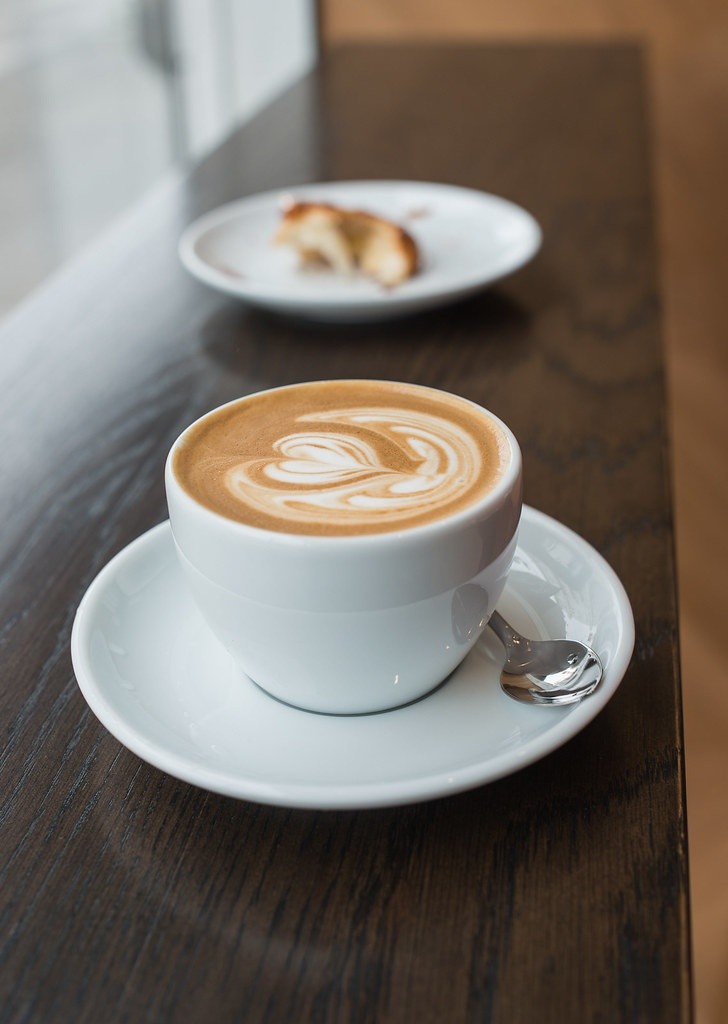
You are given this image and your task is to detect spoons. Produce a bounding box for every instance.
[487,610,604,708]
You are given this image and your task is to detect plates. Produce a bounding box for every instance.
[70,503,634,811]
[176,179,545,325]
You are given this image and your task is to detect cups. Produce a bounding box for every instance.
[164,378,523,718]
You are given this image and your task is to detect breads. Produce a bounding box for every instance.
[278,206,416,279]
